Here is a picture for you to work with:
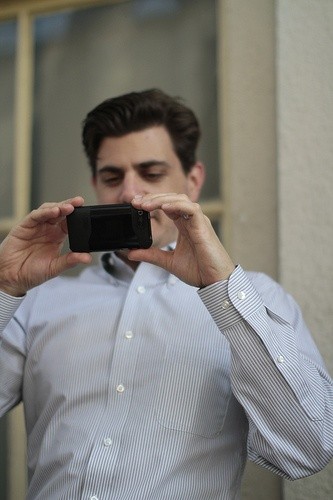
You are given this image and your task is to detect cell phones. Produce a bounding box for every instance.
[66,203,152,253]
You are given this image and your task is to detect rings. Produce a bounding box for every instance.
[183,214,190,220]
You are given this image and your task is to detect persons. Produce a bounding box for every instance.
[0,88,333,500]
